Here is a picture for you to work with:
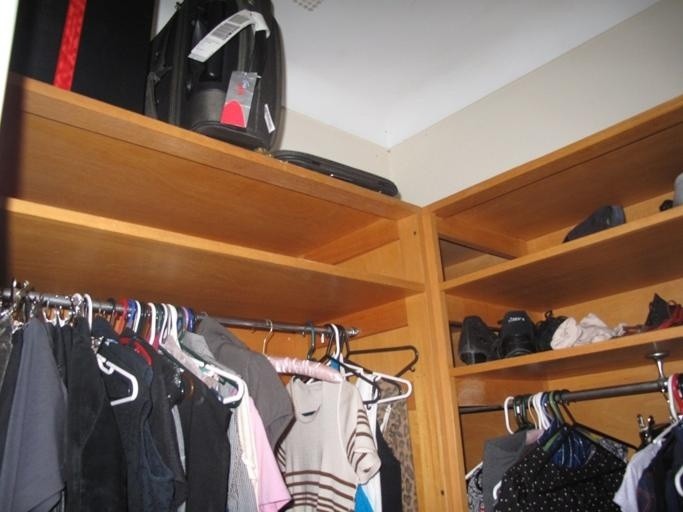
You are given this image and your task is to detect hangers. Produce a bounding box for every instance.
[466,375,681,500]
[1,276,421,417]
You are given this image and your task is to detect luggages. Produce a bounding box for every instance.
[145,0,286,154]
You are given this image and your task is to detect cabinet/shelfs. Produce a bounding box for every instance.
[1,63,469,512]
[422,88,683,511]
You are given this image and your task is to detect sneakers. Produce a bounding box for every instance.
[459,311,567,364]
